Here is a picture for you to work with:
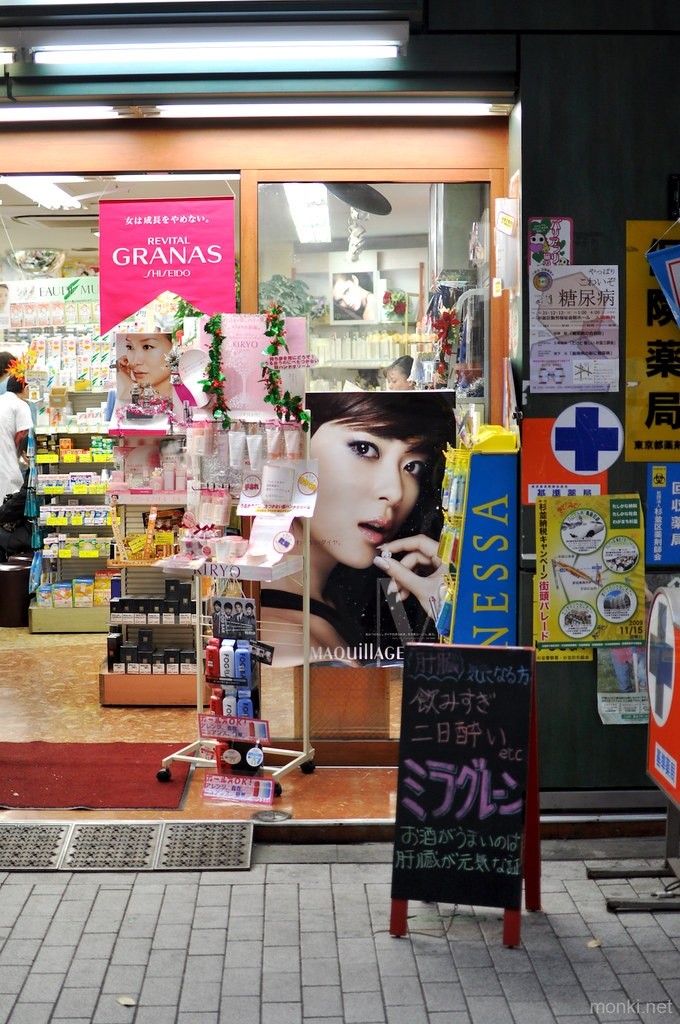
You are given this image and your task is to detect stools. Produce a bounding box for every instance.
[0,562,36,627]
[8,552,34,564]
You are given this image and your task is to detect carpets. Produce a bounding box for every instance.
[0,741,196,812]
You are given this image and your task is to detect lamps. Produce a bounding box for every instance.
[26,40,404,66]
[0,47,19,63]
[6,247,66,276]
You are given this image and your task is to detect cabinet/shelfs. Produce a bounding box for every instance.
[428,183,481,292]
[27,425,119,633]
[98,429,260,706]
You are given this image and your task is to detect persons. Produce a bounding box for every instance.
[213,600,256,640]
[0,431,38,564]
[117,333,198,411]
[0,351,16,396]
[333,273,375,320]
[383,355,425,392]
[0,284,11,328]
[0,373,34,506]
[111,495,119,505]
[259,392,456,669]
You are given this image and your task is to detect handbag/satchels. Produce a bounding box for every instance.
[1,469,32,555]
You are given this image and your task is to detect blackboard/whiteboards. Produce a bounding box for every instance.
[391,642,541,908]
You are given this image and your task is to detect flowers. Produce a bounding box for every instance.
[259,304,311,433]
[198,314,233,431]
[382,288,412,319]
[432,308,462,381]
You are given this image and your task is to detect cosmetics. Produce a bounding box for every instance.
[432,467,467,645]
[202,634,263,779]
[105,422,199,679]
[185,418,309,562]
